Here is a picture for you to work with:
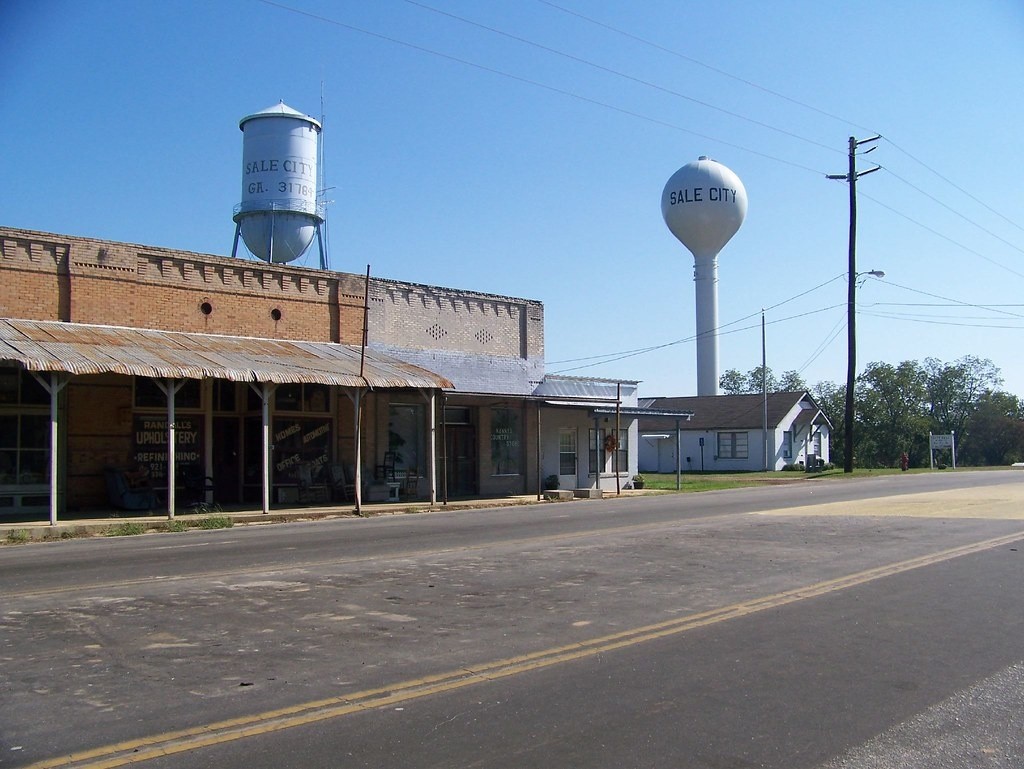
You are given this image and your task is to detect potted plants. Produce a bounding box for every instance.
[545,475,560,490]
[632,473,644,489]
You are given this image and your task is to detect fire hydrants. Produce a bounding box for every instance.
[902,452,909,471]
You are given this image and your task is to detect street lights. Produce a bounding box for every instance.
[844,270,885,473]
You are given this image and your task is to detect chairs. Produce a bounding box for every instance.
[18,451,50,485]
[294,461,329,504]
[398,465,420,500]
[374,451,396,485]
[178,464,217,509]
[329,463,355,502]
